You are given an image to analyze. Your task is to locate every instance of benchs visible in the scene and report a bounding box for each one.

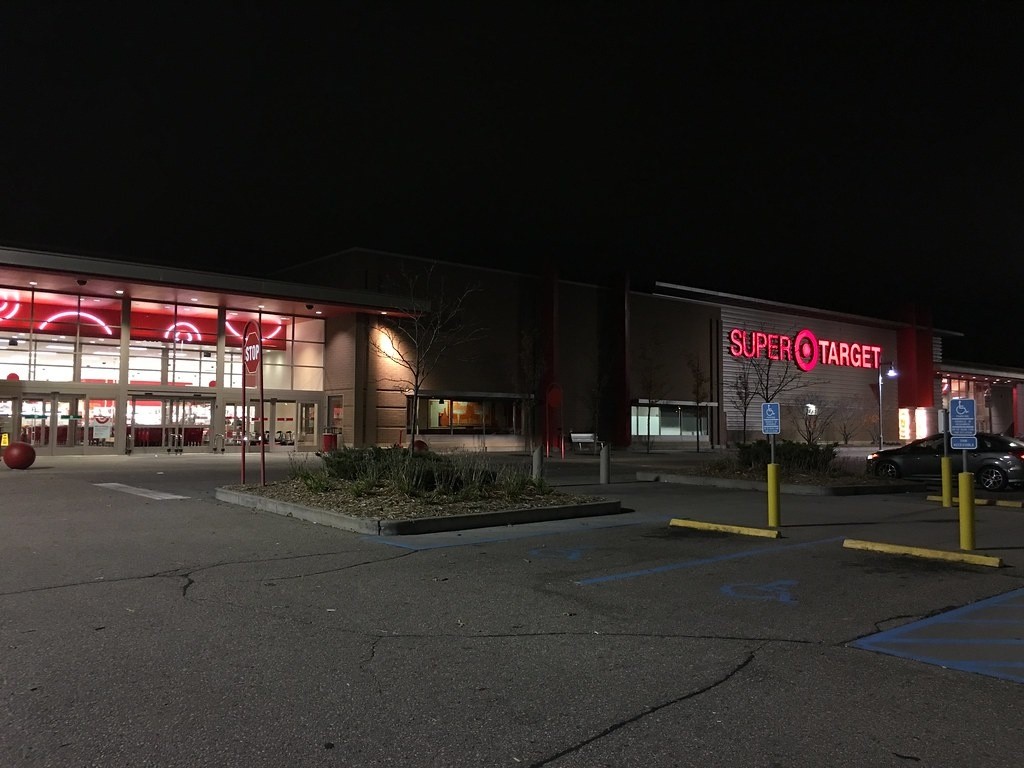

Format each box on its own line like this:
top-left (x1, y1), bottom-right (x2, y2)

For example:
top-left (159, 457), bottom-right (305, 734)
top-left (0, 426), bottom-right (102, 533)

top-left (570, 433), bottom-right (606, 453)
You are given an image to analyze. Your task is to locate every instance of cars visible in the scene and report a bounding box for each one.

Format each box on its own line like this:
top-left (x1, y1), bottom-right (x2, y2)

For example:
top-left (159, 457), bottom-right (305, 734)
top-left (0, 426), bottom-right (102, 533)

top-left (865, 431), bottom-right (1024, 492)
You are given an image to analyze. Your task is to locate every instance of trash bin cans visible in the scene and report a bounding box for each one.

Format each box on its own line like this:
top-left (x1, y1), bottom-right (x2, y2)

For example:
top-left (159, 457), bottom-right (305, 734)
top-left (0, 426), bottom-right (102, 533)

top-left (323, 426), bottom-right (339, 453)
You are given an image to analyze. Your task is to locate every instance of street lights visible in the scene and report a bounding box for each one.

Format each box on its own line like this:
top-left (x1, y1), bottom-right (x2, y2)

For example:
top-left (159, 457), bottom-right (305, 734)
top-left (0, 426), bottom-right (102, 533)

top-left (877, 361), bottom-right (898, 450)
